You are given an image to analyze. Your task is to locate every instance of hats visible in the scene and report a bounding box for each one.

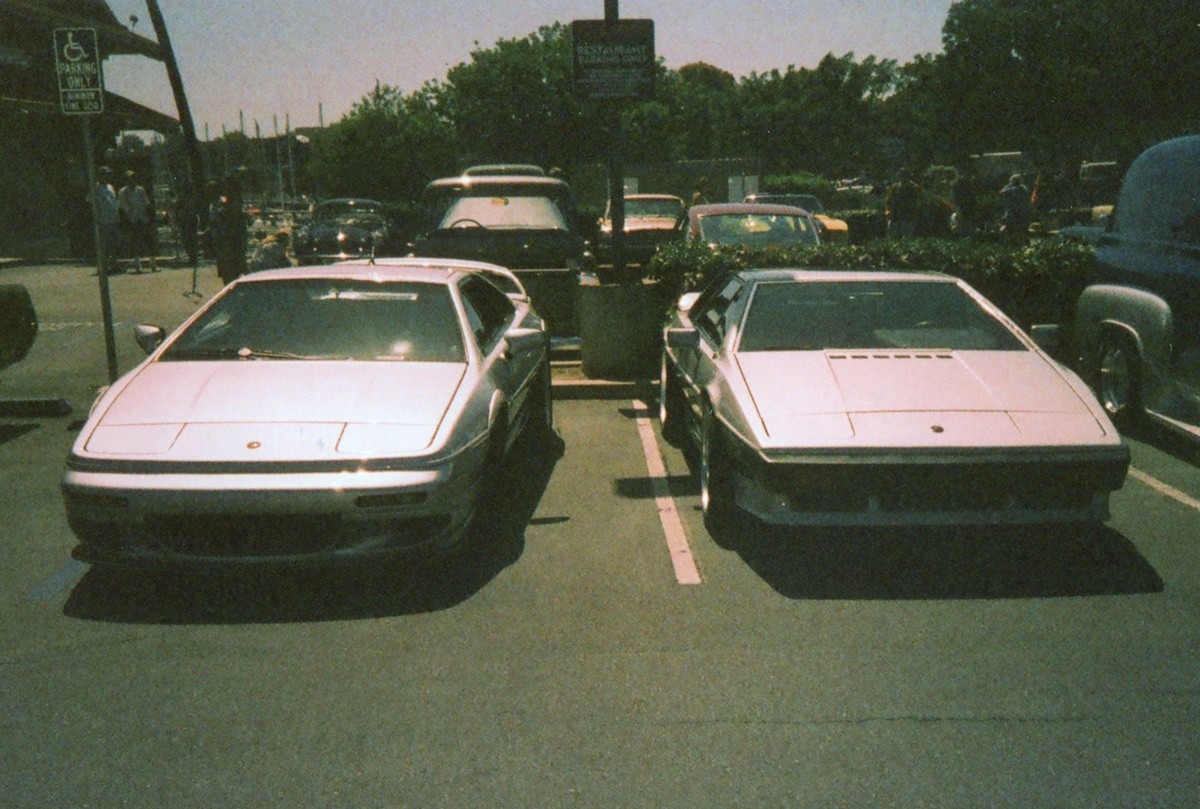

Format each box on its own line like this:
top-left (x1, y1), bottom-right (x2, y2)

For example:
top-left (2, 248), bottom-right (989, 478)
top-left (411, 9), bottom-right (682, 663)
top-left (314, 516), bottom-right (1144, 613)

top-left (96, 166), bottom-right (113, 177)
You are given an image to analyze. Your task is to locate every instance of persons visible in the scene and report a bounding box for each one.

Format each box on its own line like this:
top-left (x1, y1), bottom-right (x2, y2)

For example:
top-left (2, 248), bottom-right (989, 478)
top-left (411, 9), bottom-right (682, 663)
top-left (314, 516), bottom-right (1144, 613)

top-left (692, 175), bottom-right (713, 205)
top-left (117, 168), bottom-right (161, 274)
top-left (884, 162), bottom-right (1074, 239)
top-left (207, 198), bottom-right (292, 288)
top-left (85, 166), bottom-right (129, 276)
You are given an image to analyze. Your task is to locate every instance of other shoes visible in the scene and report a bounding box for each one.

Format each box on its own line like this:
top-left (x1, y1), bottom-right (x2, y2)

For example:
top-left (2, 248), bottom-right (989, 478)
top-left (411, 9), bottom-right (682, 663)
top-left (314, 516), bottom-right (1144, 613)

top-left (136, 267), bottom-right (142, 273)
top-left (151, 266), bottom-right (161, 272)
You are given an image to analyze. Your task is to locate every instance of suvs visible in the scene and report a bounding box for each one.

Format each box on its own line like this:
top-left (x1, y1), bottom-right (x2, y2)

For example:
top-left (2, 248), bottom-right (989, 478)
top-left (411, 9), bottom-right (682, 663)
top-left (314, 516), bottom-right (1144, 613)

top-left (1027, 134), bottom-right (1199, 443)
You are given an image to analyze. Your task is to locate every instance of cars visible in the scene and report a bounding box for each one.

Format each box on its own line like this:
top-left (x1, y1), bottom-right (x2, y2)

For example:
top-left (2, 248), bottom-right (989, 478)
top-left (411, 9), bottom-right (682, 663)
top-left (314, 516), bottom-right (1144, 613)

top-left (293, 199), bottom-right (408, 266)
top-left (413, 162), bottom-right (850, 286)
top-left (659, 268), bottom-right (1133, 550)
top-left (61, 262), bottom-right (550, 574)
